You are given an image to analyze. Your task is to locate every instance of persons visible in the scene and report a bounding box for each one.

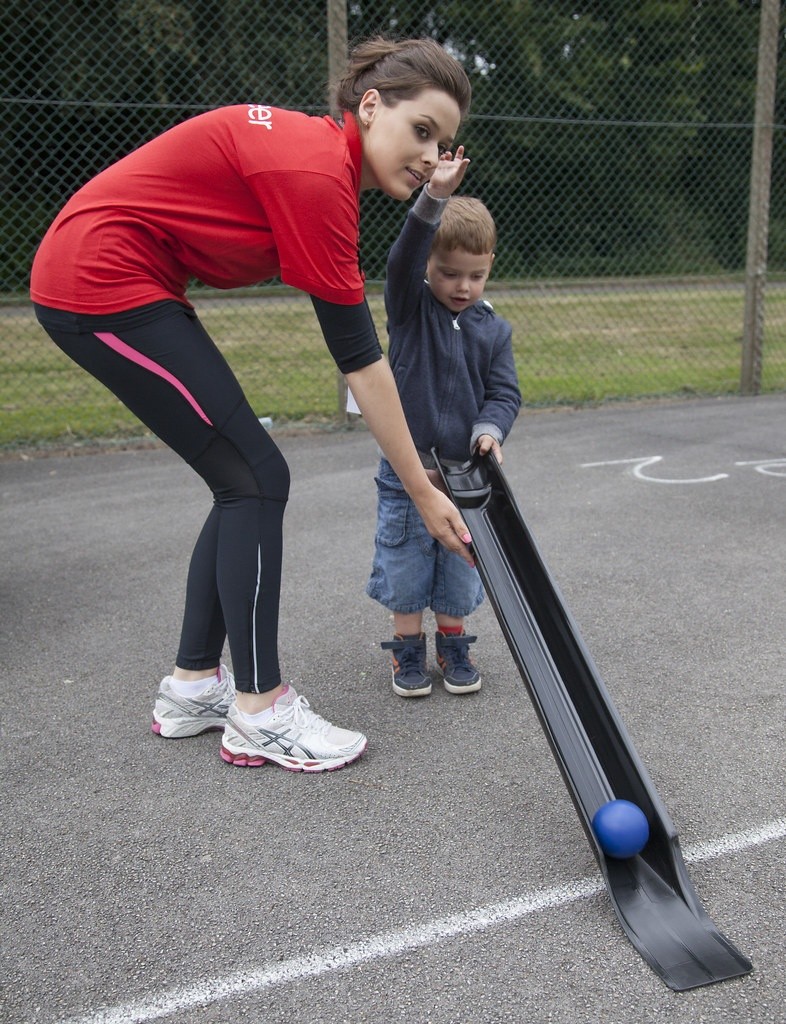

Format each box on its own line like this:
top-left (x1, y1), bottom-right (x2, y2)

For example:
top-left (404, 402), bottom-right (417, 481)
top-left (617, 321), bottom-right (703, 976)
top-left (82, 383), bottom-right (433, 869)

top-left (30, 38), bottom-right (472, 772)
top-left (365, 145), bottom-right (521, 696)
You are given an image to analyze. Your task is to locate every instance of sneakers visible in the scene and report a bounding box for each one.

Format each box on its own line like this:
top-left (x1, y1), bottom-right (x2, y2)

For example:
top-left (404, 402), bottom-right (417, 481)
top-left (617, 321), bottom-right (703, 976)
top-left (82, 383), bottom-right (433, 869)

top-left (433, 629), bottom-right (482, 694)
top-left (380, 633), bottom-right (433, 698)
top-left (151, 663), bottom-right (237, 739)
top-left (218, 685), bottom-right (368, 774)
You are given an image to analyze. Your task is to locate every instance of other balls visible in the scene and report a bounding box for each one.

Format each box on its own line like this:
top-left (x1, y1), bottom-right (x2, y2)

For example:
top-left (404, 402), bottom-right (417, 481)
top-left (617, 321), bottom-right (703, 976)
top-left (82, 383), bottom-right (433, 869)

top-left (592, 797), bottom-right (650, 859)
